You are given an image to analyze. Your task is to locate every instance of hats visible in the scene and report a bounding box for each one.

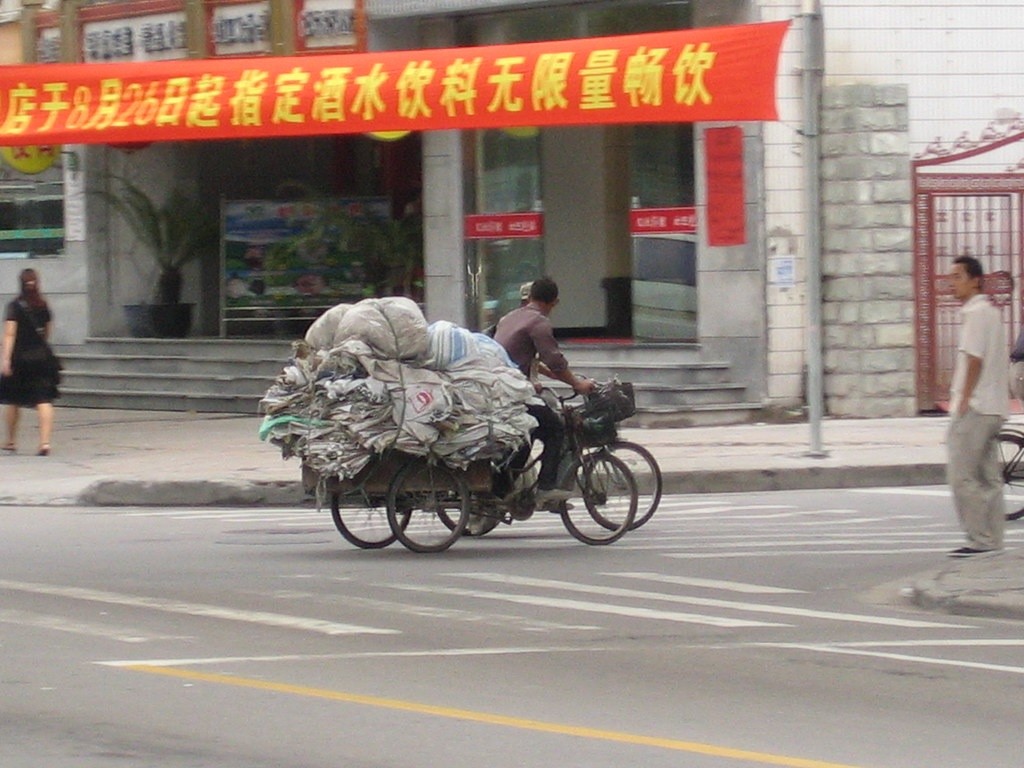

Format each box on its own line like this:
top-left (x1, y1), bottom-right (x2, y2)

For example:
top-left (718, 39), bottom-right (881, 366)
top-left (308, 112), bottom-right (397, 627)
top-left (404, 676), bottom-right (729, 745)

top-left (519, 281), bottom-right (534, 299)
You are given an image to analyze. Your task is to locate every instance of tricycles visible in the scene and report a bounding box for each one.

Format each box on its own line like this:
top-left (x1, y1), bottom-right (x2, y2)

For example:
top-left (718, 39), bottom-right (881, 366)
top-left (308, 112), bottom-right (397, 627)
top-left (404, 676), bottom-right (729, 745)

top-left (297, 370), bottom-right (639, 554)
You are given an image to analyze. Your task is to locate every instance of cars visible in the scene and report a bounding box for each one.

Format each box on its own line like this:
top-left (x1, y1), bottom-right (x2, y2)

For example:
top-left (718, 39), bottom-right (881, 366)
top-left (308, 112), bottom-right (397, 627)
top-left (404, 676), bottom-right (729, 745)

top-left (477, 233), bottom-right (699, 341)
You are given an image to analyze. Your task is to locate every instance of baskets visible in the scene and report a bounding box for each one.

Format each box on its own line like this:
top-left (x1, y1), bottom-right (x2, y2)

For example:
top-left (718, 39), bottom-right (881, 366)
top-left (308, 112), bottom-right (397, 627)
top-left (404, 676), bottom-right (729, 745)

top-left (587, 383), bottom-right (636, 421)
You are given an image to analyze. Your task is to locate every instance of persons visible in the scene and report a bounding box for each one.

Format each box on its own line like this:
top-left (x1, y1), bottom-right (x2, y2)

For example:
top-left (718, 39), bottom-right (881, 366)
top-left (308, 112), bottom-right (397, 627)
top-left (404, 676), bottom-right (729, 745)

top-left (488, 274), bottom-right (597, 511)
top-left (946, 254), bottom-right (1013, 557)
top-left (0, 268), bottom-right (63, 456)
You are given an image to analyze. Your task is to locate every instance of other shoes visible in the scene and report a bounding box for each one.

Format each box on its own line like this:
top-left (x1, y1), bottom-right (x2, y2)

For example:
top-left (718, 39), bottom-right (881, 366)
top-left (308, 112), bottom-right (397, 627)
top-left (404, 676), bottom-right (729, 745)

top-left (534, 488), bottom-right (572, 500)
top-left (947, 546), bottom-right (1006, 557)
top-left (535, 504), bottom-right (575, 511)
top-left (34, 443), bottom-right (52, 456)
top-left (3, 443), bottom-right (18, 451)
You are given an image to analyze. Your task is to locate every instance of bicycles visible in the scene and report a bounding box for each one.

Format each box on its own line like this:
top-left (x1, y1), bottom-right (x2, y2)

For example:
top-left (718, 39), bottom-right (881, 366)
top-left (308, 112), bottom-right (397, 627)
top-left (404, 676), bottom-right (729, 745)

top-left (994, 420), bottom-right (1024, 521)
top-left (435, 386), bottom-right (664, 538)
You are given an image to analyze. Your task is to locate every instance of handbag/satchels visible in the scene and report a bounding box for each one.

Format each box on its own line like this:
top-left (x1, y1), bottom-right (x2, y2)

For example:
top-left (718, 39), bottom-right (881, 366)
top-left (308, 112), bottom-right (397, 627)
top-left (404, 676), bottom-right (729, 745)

top-left (570, 396), bottom-right (618, 447)
top-left (46, 350), bottom-right (63, 385)
